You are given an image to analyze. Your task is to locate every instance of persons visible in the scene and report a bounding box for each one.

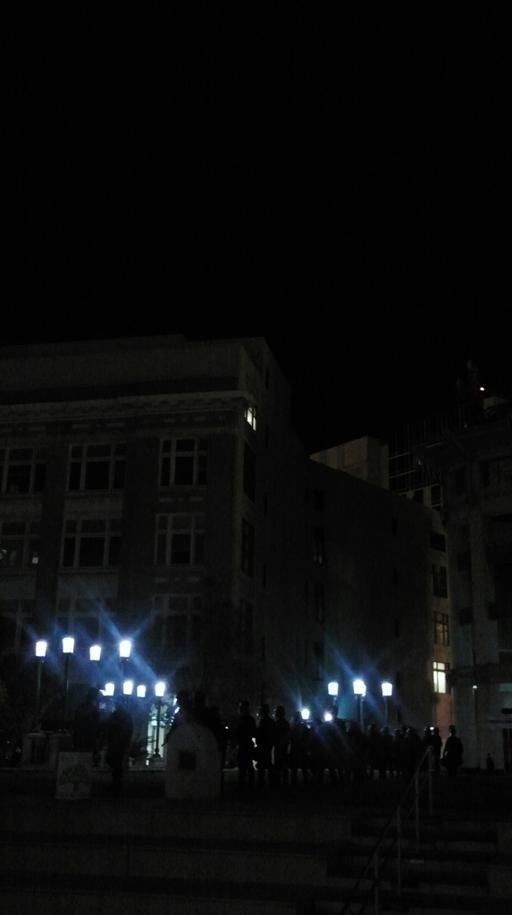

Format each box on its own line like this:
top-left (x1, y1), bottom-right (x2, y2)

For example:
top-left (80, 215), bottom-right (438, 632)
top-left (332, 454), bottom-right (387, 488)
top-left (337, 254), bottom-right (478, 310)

top-left (229, 701), bottom-right (463, 792)
top-left (486, 753), bottom-right (495, 771)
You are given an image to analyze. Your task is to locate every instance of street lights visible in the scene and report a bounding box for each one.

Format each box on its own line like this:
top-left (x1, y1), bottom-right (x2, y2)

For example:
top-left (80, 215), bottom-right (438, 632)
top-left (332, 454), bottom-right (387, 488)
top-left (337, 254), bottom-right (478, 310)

top-left (328, 680), bottom-right (339, 719)
top-left (353, 679), bottom-right (367, 726)
top-left (63, 636), bottom-right (74, 728)
top-left (381, 681), bottom-right (393, 725)
top-left (90, 637), bottom-right (165, 757)
top-left (35, 638), bottom-right (48, 729)
